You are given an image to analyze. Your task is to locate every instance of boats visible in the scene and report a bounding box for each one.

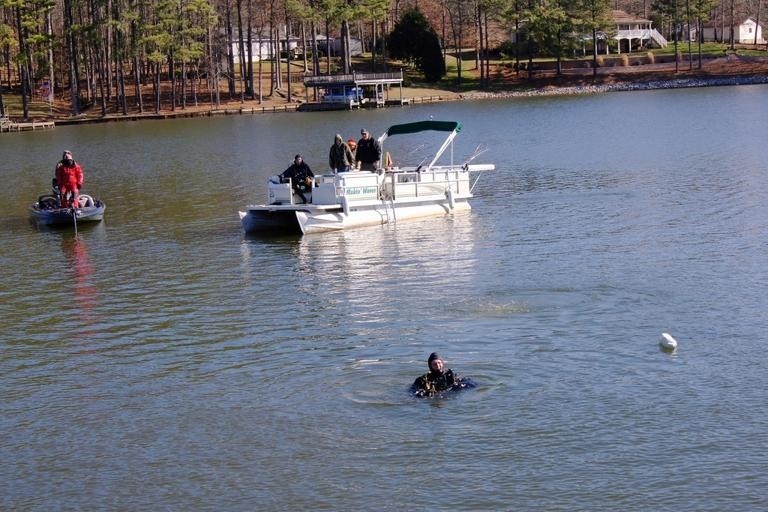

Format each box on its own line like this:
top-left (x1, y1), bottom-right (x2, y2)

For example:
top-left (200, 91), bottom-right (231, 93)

top-left (26, 193), bottom-right (109, 228)
top-left (236, 118), bottom-right (493, 239)
top-left (320, 86), bottom-right (366, 108)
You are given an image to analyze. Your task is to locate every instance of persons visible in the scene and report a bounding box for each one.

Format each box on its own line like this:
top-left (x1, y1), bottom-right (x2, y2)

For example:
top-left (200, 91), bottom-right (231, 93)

top-left (53, 149), bottom-right (84, 211)
top-left (326, 133), bottom-right (355, 174)
top-left (354, 127), bottom-right (381, 173)
top-left (279, 152), bottom-right (319, 204)
top-left (346, 137), bottom-right (358, 157)
top-left (57, 240), bottom-right (107, 354)
top-left (407, 351), bottom-right (462, 400)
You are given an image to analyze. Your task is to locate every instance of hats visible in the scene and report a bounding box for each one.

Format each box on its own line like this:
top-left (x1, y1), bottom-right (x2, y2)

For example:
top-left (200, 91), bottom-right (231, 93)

top-left (360, 127), bottom-right (368, 133)
top-left (428, 351), bottom-right (444, 376)
top-left (347, 139), bottom-right (355, 145)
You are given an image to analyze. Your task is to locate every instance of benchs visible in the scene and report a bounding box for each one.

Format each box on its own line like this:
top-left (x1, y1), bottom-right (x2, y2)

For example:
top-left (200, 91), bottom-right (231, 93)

top-left (268, 171), bottom-right (380, 205)
